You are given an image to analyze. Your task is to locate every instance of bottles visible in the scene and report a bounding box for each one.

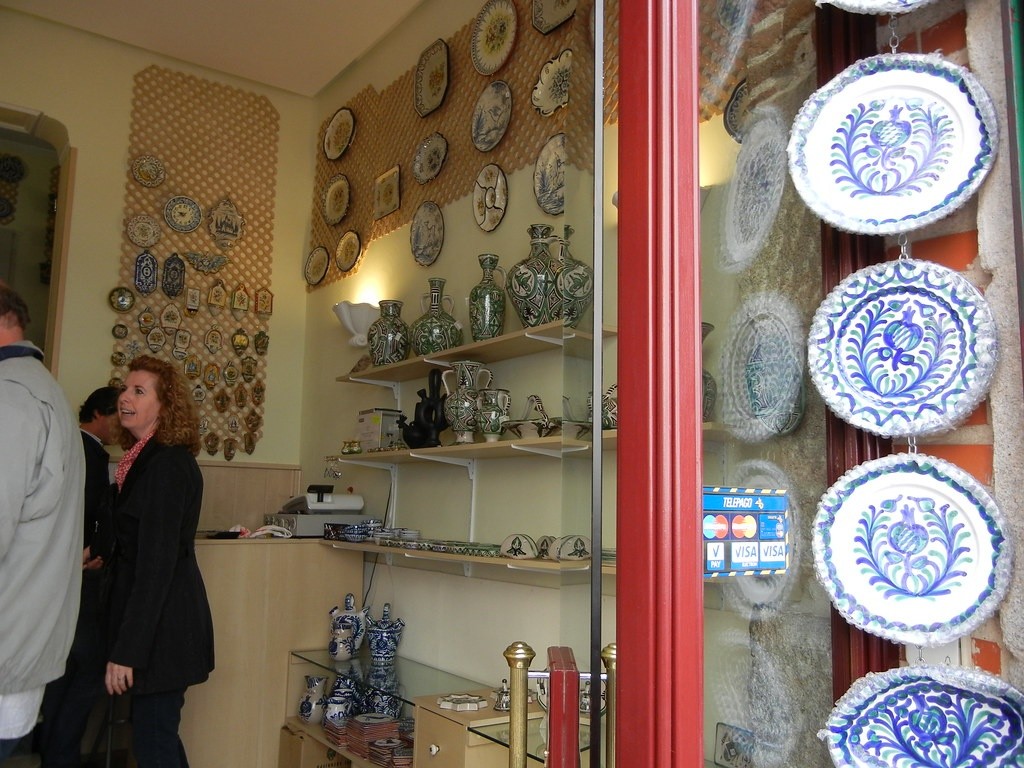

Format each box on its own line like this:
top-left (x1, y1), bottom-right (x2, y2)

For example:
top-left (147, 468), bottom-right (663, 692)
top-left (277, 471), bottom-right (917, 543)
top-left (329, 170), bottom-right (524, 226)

top-left (410, 278), bottom-right (463, 357)
top-left (368, 300), bottom-right (410, 367)
top-left (341, 440), bottom-right (361, 454)
top-left (298, 592), bottom-right (405, 724)
top-left (469, 254), bottom-right (507, 341)
top-left (441, 359), bottom-right (511, 443)
top-left (506, 224), bottom-right (594, 329)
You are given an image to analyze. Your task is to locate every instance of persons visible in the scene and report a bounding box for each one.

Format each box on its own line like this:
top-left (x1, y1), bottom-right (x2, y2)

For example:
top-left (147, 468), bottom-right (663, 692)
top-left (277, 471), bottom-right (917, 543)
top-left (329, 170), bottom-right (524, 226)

top-left (35, 385), bottom-right (126, 768)
top-left (83, 356), bottom-right (215, 768)
top-left (0, 278), bottom-right (88, 768)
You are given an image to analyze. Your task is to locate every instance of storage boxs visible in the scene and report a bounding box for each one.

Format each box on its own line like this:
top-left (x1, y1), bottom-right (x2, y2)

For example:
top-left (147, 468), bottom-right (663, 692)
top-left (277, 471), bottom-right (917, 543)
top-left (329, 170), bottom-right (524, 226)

top-left (277, 724), bottom-right (351, 768)
top-left (355, 406), bottom-right (402, 451)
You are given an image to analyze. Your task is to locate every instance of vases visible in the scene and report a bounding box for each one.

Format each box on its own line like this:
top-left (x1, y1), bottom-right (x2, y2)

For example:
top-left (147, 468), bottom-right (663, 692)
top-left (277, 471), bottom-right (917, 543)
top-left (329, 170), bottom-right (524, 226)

top-left (701, 321), bottom-right (717, 422)
top-left (560, 225), bottom-right (593, 330)
top-left (474, 386), bottom-right (511, 444)
top-left (332, 687), bottom-right (361, 717)
top-left (323, 697), bottom-right (353, 725)
top-left (365, 300), bottom-right (411, 367)
top-left (407, 277), bottom-right (464, 357)
top-left (296, 674), bottom-right (330, 725)
top-left (442, 359), bottom-right (494, 445)
top-left (468, 253), bottom-right (506, 341)
top-left (506, 222), bottom-right (563, 330)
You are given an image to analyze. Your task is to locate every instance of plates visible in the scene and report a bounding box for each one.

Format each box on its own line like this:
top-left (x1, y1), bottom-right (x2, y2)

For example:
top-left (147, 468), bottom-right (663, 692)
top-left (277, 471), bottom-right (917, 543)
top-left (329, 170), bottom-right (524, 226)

top-left (164, 196), bottom-right (203, 233)
top-left (127, 214), bottom-right (160, 247)
top-left (106, 285), bottom-right (135, 313)
top-left (304, 38), bottom-right (450, 285)
top-left (470, 0), bottom-right (578, 233)
top-left (132, 156), bottom-right (165, 187)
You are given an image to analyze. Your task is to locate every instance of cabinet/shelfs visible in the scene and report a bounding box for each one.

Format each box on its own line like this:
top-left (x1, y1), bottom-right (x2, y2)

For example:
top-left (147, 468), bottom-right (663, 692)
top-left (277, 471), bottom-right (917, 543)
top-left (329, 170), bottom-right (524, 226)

top-left (600, 420), bottom-right (727, 590)
top-left (562, 327), bottom-right (602, 587)
top-left (325, 320), bottom-right (562, 589)
top-left (602, 322), bottom-right (616, 579)
top-left (287, 648), bottom-right (591, 768)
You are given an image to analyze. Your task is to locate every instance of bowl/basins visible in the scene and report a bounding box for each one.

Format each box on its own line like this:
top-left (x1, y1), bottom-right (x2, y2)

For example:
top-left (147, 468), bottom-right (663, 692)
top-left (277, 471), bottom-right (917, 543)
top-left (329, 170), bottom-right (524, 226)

top-left (323, 519), bottom-right (501, 558)
